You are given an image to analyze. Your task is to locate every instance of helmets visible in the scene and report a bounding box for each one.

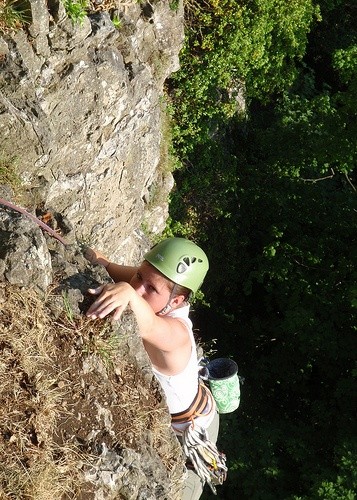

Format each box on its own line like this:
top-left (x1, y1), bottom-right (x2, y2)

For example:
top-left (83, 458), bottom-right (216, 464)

top-left (143, 237), bottom-right (209, 299)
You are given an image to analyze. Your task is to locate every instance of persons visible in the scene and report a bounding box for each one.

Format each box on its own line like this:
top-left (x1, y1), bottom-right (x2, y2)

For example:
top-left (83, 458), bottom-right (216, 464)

top-left (81, 237), bottom-right (220, 488)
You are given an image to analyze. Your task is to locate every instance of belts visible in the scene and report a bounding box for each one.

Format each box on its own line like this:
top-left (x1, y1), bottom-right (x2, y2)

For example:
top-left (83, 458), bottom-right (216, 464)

top-left (169, 385), bottom-right (210, 425)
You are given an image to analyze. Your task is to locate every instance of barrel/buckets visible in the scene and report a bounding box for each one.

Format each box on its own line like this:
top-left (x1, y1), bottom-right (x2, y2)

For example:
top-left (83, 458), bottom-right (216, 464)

top-left (205, 357), bottom-right (241, 414)
